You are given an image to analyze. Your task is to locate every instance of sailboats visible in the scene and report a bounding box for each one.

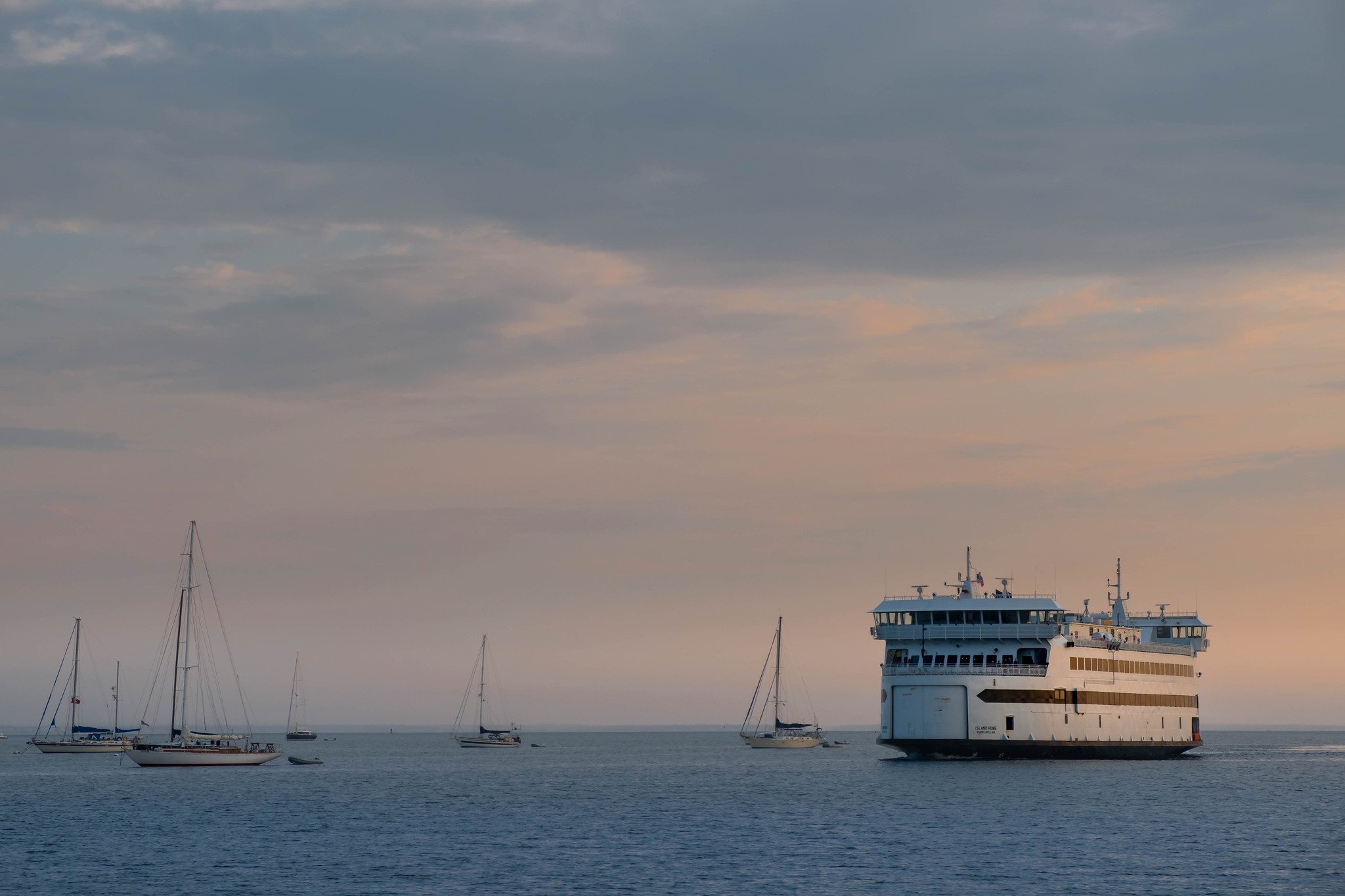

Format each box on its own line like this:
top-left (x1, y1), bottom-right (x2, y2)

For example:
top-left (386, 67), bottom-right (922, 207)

top-left (739, 609), bottom-right (829, 748)
top-left (119, 516), bottom-right (283, 767)
top-left (447, 633), bottom-right (523, 748)
top-left (11, 616), bottom-right (141, 755)
top-left (284, 650), bottom-right (318, 742)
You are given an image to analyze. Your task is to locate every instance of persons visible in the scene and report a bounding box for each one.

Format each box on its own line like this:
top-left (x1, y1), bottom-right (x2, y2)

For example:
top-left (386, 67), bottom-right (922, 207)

top-left (896, 650), bottom-right (902, 664)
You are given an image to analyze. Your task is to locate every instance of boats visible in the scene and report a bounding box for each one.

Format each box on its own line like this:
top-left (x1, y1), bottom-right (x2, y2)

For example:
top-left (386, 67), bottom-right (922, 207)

top-left (287, 755), bottom-right (325, 765)
top-left (324, 738), bottom-right (337, 741)
top-left (865, 545), bottom-right (1215, 762)
top-left (0, 733), bottom-right (8, 739)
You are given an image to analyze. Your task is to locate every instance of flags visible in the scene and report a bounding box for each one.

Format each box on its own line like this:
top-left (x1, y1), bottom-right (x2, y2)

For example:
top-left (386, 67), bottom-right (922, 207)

top-left (977, 574), bottom-right (984, 587)
top-left (70, 697), bottom-right (80, 704)
top-left (141, 720), bottom-right (150, 727)
